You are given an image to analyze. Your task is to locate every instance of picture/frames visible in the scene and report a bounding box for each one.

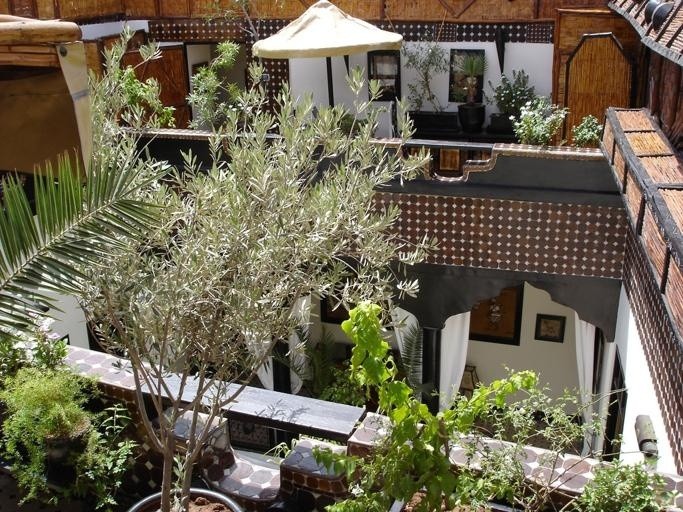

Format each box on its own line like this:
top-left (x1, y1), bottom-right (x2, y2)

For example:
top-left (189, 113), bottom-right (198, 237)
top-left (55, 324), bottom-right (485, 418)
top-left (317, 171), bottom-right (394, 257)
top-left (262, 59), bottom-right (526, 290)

top-left (534, 313), bottom-right (566, 344)
top-left (468, 281), bottom-right (523, 347)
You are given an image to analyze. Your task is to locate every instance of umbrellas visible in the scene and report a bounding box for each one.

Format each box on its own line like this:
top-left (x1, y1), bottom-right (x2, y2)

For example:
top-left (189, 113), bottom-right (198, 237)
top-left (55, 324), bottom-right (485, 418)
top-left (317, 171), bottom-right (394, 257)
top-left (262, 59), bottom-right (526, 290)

top-left (250, 1), bottom-right (404, 118)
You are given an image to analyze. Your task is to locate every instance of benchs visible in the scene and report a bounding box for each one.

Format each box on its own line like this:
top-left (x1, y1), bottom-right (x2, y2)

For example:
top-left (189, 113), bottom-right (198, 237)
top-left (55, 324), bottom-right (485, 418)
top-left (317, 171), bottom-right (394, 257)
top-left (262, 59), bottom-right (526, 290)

top-left (405, 111), bottom-right (517, 142)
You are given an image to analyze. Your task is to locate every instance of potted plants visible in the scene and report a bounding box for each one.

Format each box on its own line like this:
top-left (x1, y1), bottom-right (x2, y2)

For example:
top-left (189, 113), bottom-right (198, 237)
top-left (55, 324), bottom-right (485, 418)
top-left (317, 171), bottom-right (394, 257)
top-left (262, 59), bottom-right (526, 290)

top-left (456, 51), bottom-right (489, 132)
top-left (74, 62), bottom-right (432, 512)
top-left (311, 297), bottom-right (524, 512)
